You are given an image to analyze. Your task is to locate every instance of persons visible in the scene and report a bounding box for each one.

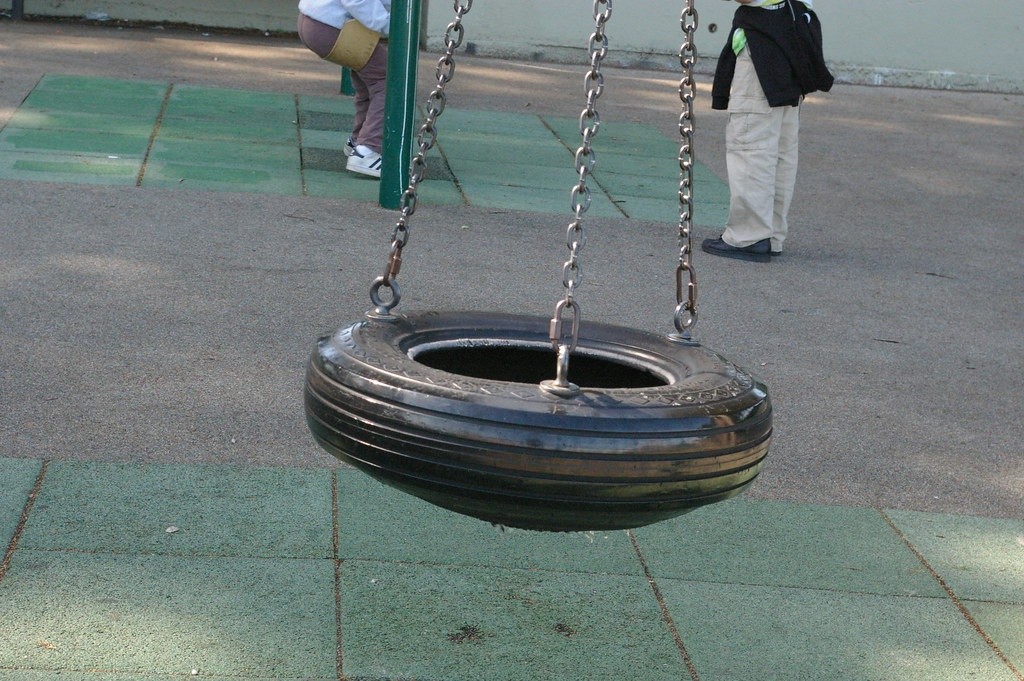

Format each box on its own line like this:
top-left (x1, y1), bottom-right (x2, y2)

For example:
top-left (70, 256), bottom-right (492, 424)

top-left (701, 0), bottom-right (835, 264)
top-left (297, 0), bottom-right (392, 179)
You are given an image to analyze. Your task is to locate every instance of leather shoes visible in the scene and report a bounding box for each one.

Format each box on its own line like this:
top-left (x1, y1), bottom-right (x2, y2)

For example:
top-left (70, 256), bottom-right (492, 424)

top-left (701, 234), bottom-right (772, 263)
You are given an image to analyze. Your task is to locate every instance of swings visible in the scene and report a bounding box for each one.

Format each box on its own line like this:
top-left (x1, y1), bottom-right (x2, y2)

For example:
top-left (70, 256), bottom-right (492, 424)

top-left (295, 1), bottom-right (777, 537)
top-left (319, 18), bottom-right (382, 73)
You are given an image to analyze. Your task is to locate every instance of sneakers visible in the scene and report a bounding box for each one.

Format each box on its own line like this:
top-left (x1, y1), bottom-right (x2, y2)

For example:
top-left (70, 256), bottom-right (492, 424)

top-left (343, 135), bottom-right (359, 157)
top-left (345, 141), bottom-right (382, 177)
